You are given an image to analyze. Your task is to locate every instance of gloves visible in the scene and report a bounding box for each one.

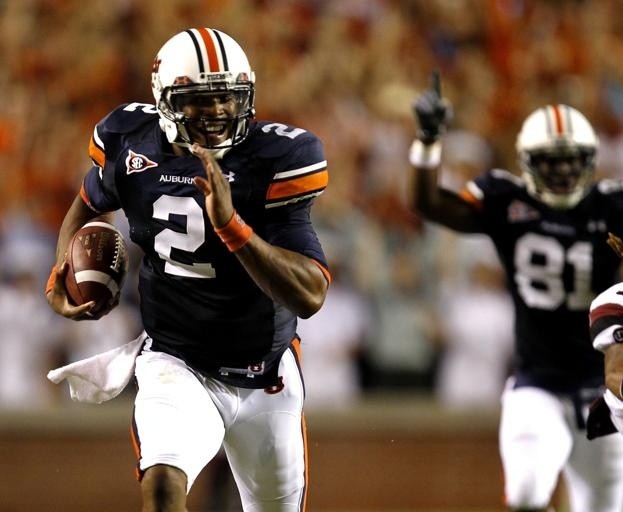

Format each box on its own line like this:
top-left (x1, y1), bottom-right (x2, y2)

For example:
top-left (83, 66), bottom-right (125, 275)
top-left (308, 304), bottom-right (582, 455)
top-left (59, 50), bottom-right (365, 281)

top-left (412, 68), bottom-right (452, 145)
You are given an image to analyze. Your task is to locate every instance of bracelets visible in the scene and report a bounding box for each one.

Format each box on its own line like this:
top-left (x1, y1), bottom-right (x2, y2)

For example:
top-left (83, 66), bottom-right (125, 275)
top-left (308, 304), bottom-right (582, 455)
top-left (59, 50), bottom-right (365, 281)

top-left (214, 210), bottom-right (254, 252)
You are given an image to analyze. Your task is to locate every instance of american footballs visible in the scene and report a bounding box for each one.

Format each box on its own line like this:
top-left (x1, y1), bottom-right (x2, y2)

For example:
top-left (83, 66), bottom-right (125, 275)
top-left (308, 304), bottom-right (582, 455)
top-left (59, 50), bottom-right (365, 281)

top-left (64, 216), bottom-right (130, 319)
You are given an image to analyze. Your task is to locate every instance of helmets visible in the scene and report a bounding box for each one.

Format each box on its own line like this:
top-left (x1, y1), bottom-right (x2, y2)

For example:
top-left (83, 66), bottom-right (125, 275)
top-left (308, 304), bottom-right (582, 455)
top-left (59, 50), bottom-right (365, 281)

top-left (150, 26), bottom-right (258, 152)
top-left (514, 103), bottom-right (600, 209)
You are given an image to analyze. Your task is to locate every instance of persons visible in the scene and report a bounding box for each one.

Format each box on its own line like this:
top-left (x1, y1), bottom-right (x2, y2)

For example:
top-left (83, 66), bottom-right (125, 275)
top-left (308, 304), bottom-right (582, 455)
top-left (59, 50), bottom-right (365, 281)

top-left (395, 64), bottom-right (622, 509)
top-left (42, 23), bottom-right (328, 512)
top-left (0, 0), bottom-right (623, 403)
